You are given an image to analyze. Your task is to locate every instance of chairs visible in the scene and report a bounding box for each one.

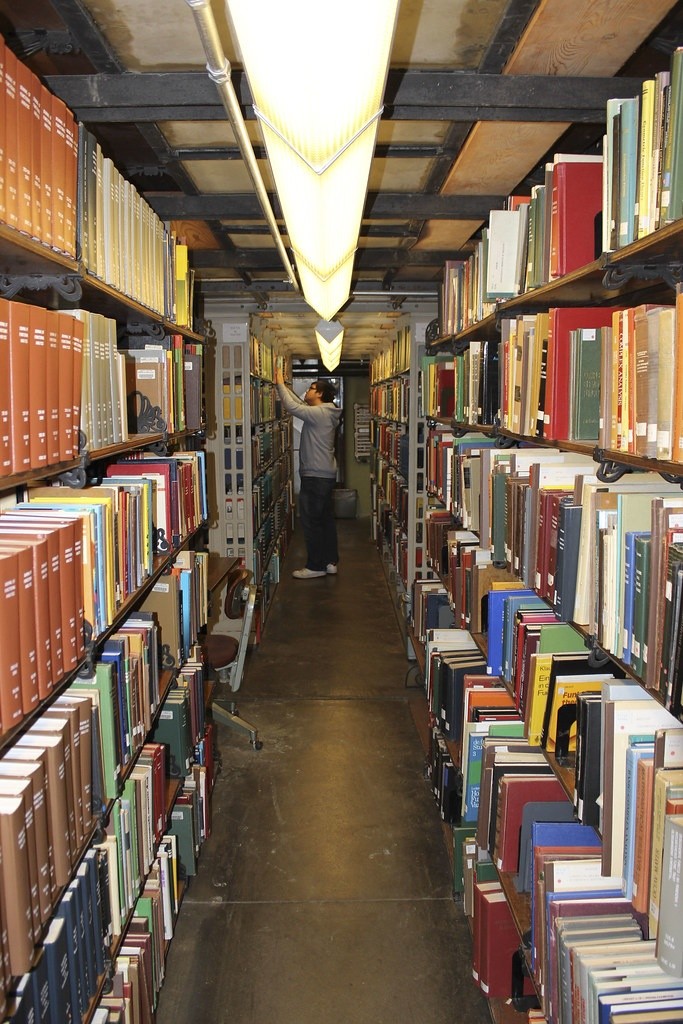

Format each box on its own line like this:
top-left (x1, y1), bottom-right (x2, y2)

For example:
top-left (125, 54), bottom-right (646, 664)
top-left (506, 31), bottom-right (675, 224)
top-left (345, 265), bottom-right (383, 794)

top-left (202, 564), bottom-right (262, 749)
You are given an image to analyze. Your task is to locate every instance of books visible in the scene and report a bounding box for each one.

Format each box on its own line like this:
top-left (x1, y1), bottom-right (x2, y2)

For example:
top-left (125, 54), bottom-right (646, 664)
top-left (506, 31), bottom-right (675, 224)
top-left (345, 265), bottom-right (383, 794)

top-left (0, 32), bottom-right (293, 1024)
top-left (371, 48), bottom-right (683, 1024)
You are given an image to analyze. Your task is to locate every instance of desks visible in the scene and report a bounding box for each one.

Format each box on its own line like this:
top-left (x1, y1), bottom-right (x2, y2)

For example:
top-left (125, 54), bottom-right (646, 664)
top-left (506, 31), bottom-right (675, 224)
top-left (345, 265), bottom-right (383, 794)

top-left (206, 556), bottom-right (240, 594)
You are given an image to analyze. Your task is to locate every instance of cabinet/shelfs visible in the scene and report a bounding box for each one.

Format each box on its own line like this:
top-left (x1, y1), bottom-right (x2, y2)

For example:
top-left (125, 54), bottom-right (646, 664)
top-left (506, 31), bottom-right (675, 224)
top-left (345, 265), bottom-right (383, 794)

top-left (210, 310), bottom-right (295, 646)
top-left (366, 311), bottom-right (440, 658)
top-left (424, 217), bottom-right (683, 1024)
top-left (0, 221), bottom-right (219, 1023)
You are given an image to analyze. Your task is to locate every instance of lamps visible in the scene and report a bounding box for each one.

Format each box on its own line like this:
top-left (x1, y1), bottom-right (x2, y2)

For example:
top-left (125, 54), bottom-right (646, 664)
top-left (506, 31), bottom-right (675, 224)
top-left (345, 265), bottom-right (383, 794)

top-left (314, 316), bottom-right (344, 372)
top-left (223, 0), bottom-right (396, 321)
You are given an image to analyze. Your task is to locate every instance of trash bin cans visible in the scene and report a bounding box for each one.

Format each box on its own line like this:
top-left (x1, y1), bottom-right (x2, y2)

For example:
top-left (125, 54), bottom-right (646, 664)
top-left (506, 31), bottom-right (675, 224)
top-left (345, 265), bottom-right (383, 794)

top-left (332, 487), bottom-right (359, 520)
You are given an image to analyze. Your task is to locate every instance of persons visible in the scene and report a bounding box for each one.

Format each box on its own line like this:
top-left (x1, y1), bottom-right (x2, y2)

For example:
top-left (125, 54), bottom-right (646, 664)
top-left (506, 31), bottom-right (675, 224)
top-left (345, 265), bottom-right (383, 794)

top-left (277, 367), bottom-right (343, 578)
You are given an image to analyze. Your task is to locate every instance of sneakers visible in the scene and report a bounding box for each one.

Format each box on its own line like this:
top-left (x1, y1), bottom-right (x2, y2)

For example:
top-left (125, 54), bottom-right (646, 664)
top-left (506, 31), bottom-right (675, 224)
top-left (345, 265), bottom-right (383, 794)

top-left (292, 568), bottom-right (326, 579)
top-left (326, 564), bottom-right (337, 573)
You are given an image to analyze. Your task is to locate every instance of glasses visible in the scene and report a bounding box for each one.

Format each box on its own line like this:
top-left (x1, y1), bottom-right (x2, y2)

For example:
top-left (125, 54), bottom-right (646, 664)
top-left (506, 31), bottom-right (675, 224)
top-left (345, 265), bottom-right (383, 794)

top-left (309, 387), bottom-right (317, 391)
